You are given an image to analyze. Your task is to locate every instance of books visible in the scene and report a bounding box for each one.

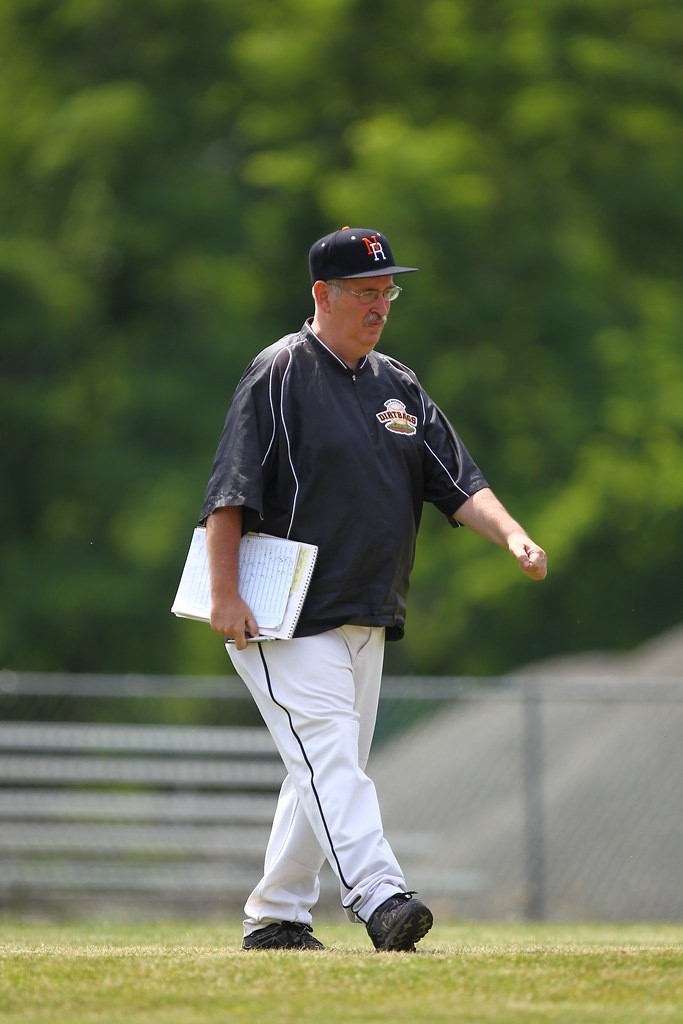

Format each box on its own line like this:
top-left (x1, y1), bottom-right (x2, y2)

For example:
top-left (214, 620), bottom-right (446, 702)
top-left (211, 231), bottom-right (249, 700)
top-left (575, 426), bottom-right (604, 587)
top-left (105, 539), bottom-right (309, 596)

top-left (171, 526), bottom-right (318, 641)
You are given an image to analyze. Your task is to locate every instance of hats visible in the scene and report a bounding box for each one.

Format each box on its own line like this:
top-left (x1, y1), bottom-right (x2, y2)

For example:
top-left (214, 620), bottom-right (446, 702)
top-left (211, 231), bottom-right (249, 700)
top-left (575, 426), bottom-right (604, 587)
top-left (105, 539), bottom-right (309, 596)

top-left (309, 226), bottom-right (419, 285)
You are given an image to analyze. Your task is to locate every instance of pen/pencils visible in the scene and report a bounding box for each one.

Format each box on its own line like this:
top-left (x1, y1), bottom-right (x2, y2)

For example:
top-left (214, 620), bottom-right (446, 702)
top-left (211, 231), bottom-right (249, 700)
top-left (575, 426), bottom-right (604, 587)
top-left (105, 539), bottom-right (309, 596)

top-left (225, 637), bottom-right (280, 642)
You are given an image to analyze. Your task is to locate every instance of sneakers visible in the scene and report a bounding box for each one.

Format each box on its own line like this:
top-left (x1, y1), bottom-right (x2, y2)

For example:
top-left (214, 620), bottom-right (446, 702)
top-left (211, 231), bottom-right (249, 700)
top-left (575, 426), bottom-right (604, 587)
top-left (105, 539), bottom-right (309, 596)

top-left (364, 890), bottom-right (433, 952)
top-left (241, 921), bottom-right (326, 950)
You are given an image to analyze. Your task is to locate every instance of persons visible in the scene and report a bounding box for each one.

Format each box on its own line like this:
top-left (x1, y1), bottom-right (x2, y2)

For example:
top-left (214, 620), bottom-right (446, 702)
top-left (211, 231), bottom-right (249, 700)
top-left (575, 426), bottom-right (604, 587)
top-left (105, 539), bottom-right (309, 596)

top-left (197, 226), bottom-right (549, 953)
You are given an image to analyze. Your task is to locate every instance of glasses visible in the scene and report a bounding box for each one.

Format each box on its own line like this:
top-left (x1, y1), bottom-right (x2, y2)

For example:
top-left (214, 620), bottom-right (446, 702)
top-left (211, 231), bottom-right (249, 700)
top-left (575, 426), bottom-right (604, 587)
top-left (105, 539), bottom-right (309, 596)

top-left (326, 283), bottom-right (403, 304)
